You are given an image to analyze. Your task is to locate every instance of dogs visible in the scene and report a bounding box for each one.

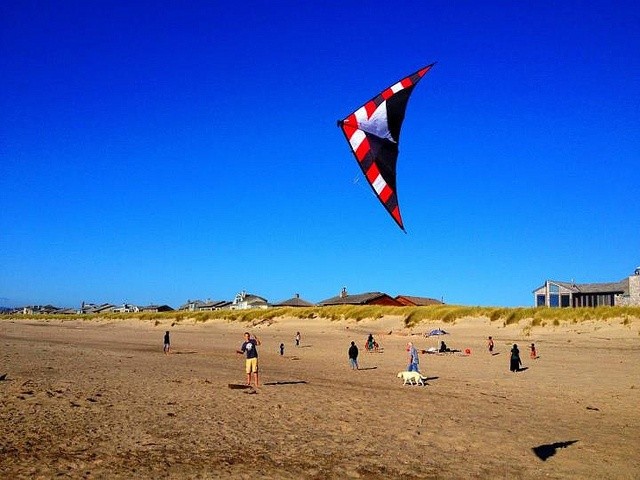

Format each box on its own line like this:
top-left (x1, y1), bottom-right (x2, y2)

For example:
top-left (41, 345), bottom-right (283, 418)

top-left (397, 371), bottom-right (428, 386)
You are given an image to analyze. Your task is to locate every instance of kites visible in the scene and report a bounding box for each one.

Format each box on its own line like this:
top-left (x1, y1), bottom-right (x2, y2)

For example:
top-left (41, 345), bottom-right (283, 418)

top-left (337, 61), bottom-right (437, 236)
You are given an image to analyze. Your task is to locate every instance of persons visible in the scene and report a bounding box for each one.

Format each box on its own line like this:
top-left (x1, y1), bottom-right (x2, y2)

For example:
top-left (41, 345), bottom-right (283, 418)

top-left (439, 341), bottom-right (447, 352)
top-left (406, 343), bottom-right (419, 374)
top-left (295, 332), bottom-right (301, 346)
top-left (487, 336), bottom-right (495, 353)
top-left (236, 333), bottom-right (260, 388)
top-left (162, 331), bottom-right (170, 354)
top-left (348, 341), bottom-right (359, 370)
top-left (510, 344), bottom-right (522, 372)
top-left (278, 343), bottom-right (285, 355)
top-left (365, 333), bottom-right (379, 353)
top-left (528, 343), bottom-right (536, 359)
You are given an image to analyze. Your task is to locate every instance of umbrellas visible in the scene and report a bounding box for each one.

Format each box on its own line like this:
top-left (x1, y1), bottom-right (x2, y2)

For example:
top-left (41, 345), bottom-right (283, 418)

top-left (429, 328), bottom-right (449, 354)
